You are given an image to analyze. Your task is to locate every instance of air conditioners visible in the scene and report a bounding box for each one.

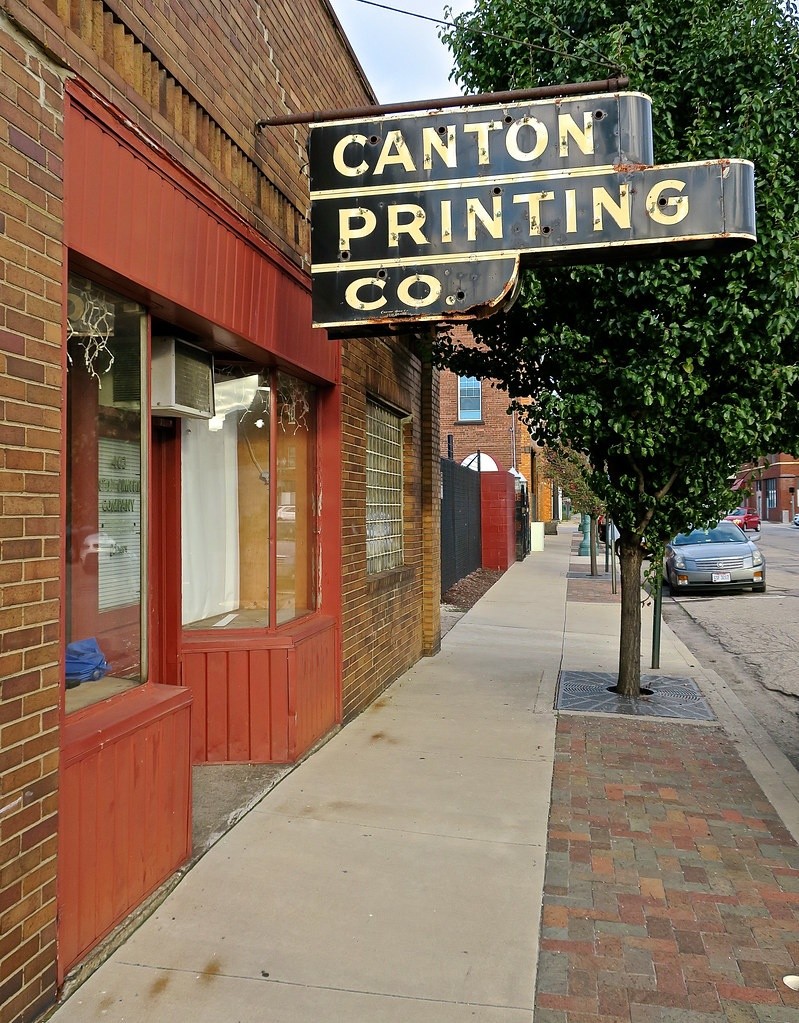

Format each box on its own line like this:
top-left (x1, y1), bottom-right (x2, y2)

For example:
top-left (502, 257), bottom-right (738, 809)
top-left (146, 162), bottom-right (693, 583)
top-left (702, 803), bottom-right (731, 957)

top-left (99, 332), bottom-right (216, 420)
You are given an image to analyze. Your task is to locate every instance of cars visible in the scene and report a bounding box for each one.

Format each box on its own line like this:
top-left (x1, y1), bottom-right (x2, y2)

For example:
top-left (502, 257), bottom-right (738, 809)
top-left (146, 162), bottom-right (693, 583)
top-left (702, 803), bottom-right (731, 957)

top-left (663, 520), bottom-right (766, 596)
top-left (724, 506), bottom-right (761, 532)
top-left (794, 513), bottom-right (799, 526)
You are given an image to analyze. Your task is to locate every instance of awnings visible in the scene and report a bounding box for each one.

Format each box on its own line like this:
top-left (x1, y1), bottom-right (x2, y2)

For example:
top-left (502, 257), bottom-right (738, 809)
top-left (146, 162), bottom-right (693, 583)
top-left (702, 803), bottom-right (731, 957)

top-left (730, 479), bottom-right (745, 491)
